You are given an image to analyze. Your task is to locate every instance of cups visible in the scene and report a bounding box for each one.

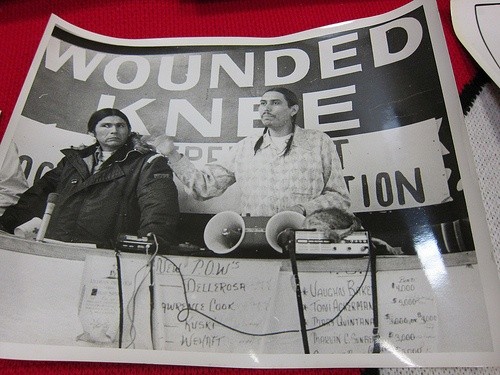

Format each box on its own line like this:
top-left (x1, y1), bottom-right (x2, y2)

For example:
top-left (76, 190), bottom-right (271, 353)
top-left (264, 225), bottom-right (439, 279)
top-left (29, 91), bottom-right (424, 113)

top-left (418, 218), bottom-right (475, 256)
top-left (13, 217), bottom-right (42, 239)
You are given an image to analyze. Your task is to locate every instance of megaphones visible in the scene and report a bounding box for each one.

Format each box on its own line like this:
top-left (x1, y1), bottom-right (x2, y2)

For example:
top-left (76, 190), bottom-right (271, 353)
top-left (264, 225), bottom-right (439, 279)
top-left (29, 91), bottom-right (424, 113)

top-left (203, 212), bottom-right (245, 254)
top-left (265, 211), bottom-right (308, 253)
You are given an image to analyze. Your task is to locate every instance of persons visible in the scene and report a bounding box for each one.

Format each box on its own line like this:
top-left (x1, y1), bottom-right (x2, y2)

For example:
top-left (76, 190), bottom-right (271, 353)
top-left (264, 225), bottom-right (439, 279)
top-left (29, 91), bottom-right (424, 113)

top-left (0, 107), bottom-right (179, 247)
top-left (156, 86), bottom-right (353, 217)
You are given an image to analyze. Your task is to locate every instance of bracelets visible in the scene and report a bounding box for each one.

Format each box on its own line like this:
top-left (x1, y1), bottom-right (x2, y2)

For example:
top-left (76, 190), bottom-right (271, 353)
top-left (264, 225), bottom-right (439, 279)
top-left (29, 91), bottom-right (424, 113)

top-left (294, 204), bottom-right (306, 216)
